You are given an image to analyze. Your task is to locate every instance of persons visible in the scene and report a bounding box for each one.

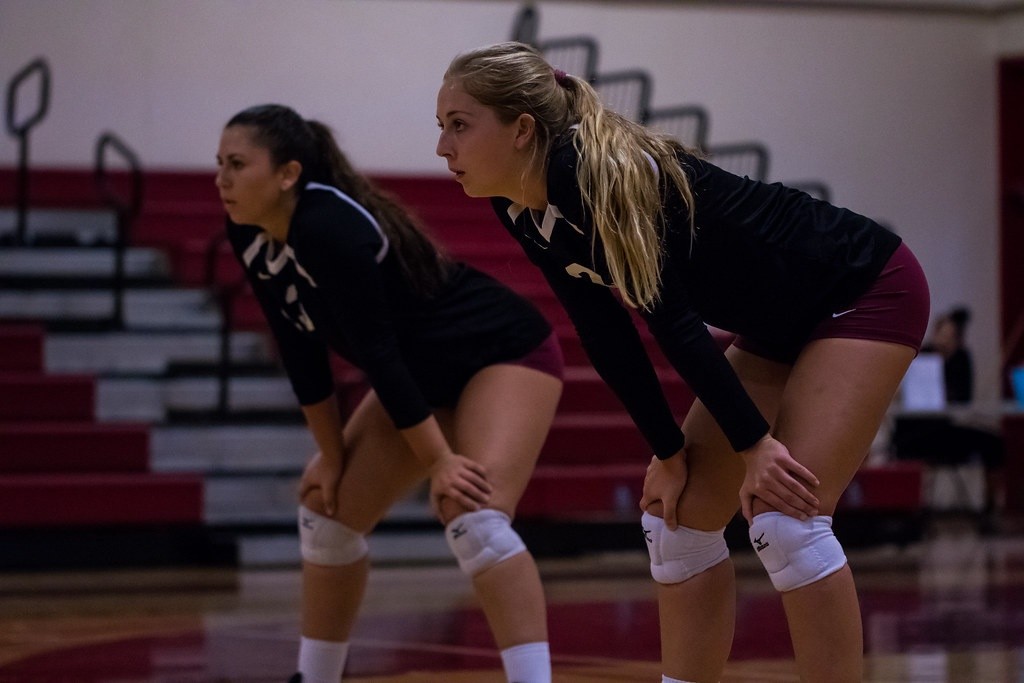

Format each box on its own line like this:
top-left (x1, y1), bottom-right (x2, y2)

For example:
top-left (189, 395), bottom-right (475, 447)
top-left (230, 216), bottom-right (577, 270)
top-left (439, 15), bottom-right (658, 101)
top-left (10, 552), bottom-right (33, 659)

top-left (436, 42), bottom-right (931, 683)
top-left (216, 105), bottom-right (565, 683)
top-left (918, 310), bottom-right (974, 407)
